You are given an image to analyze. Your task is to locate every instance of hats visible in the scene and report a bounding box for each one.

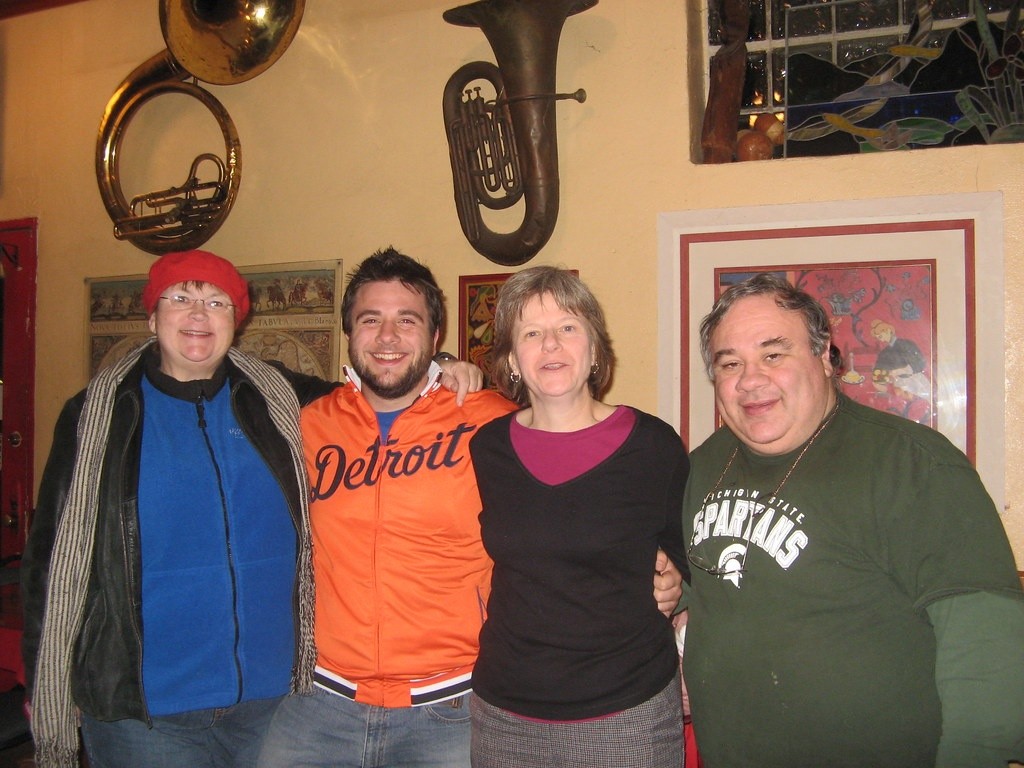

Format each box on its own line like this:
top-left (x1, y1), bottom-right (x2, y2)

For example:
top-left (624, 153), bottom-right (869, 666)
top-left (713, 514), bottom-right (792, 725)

top-left (144, 250), bottom-right (250, 330)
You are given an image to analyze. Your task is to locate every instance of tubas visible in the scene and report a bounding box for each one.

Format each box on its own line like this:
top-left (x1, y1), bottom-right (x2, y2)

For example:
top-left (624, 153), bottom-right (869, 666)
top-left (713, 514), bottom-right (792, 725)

top-left (442, 0), bottom-right (600, 267)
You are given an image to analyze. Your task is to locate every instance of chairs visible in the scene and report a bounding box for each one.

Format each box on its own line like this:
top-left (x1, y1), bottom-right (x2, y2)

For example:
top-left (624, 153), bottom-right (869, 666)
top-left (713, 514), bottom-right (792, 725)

top-left (0, 554), bottom-right (84, 768)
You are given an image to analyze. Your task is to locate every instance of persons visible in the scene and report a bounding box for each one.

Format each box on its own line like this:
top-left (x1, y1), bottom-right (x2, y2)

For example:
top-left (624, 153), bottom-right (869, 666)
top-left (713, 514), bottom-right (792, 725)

top-left (670, 273), bottom-right (1024, 768)
top-left (468, 266), bottom-right (691, 768)
top-left (256, 245), bottom-right (682, 768)
top-left (20, 251), bottom-right (482, 768)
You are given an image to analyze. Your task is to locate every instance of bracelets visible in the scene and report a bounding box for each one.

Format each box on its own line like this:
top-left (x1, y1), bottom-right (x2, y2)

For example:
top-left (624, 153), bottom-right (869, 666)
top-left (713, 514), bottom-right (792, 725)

top-left (441, 357), bottom-right (452, 360)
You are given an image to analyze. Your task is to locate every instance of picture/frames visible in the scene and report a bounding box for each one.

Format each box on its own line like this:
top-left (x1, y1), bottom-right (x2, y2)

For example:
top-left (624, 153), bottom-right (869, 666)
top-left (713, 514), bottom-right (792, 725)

top-left (657, 192), bottom-right (1006, 515)
top-left (459, 270), bottom-right (578, 406)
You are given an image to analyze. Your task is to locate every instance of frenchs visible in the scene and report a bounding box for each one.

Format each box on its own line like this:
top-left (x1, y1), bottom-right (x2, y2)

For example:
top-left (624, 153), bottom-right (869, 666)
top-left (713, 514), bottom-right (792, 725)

top-left (95, 0), bottom-right (306, 257)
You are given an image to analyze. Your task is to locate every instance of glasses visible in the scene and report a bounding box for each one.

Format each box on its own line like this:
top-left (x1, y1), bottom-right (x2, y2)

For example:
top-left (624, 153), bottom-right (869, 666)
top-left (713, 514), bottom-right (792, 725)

top-left (687, 482), bottom-right (774, 577)
top-left (160, 295), bottom-right (236, 314)
